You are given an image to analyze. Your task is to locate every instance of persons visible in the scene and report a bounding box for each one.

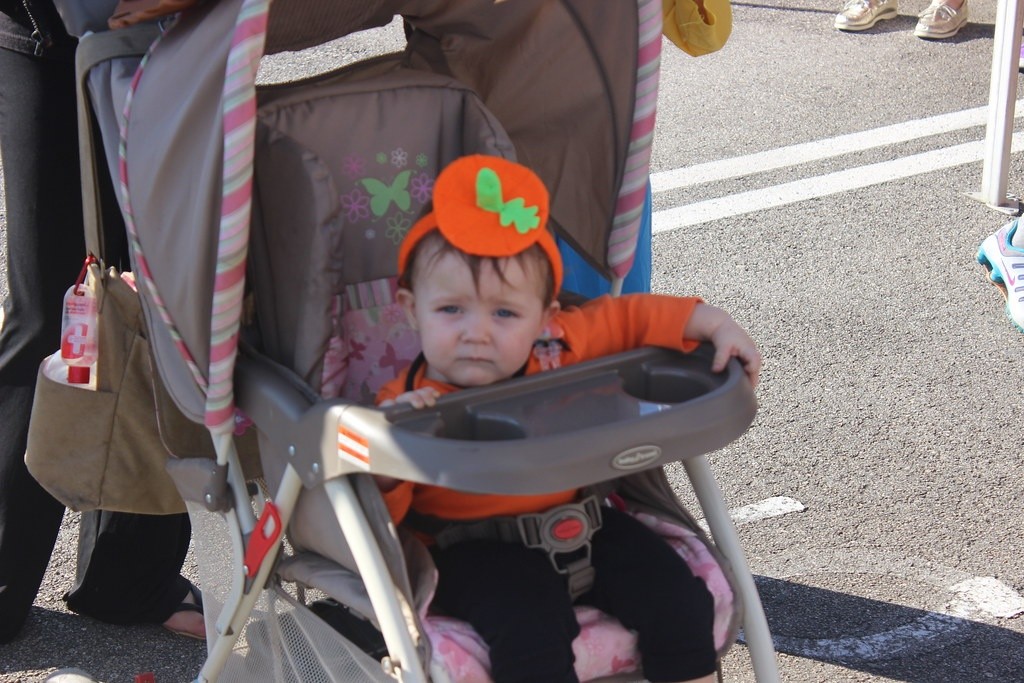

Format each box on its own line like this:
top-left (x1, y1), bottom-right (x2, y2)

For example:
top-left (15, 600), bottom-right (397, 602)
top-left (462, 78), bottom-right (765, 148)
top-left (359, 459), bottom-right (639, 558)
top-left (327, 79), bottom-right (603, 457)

top-left (2, 0), bottom-right (226, 656)
top-left (977, 208), bottom-right (1024, 336)
top-left (374, 157), bottom-right (762, 681)
top-left (834, 1), bottom-right (970, 38)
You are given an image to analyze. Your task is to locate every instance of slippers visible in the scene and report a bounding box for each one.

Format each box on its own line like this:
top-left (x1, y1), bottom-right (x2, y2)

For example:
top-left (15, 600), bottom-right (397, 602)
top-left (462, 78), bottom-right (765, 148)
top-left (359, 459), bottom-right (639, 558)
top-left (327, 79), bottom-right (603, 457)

top-left (160, 583), bottom-right (223, 640)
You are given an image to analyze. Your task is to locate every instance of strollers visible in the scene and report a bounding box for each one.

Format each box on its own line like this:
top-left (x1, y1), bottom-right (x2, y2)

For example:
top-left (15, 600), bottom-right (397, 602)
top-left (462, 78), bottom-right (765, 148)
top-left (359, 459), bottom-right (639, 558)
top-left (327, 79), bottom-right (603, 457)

top-left (55, 0), bottom-right (784, 682)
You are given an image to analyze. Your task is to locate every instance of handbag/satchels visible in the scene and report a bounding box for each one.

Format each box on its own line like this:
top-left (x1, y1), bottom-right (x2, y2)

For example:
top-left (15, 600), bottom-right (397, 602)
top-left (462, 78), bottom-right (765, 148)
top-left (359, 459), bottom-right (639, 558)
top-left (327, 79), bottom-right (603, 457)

top-left (23, 264), bottom-right (264, 515)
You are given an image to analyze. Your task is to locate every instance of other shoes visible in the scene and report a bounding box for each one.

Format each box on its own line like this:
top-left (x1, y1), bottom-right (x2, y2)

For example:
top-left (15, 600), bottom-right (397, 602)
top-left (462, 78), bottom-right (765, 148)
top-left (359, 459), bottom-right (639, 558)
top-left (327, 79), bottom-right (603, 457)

top-left (1019, 42), bottom-right (1024, 69)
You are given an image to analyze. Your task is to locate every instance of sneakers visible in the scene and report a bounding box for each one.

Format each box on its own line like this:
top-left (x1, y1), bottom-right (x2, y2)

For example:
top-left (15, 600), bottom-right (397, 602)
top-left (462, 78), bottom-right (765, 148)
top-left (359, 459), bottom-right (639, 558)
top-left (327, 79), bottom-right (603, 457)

top-left (977, 220), bottom-right (1024, 333)
top-left (913, 0), bottom-right (967, 38)
top-left (834, 0), bottom-right (897, 30)
